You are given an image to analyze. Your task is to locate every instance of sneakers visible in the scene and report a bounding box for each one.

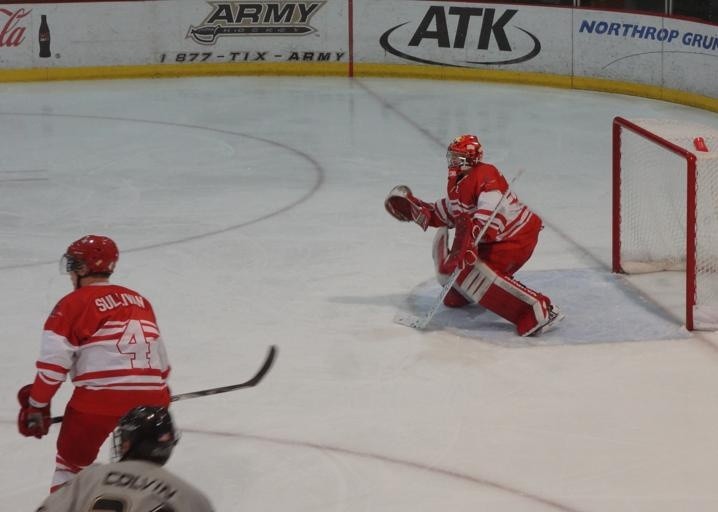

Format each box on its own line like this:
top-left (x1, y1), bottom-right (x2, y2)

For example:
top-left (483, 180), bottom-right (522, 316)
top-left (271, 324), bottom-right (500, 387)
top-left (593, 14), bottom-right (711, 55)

top-left (517, 292), bottom-right (553, 336)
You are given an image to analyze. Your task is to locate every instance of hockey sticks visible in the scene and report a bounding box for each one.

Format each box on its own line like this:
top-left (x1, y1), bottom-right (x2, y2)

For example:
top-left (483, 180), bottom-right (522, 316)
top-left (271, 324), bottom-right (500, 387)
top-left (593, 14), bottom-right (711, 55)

top-left (392, 166), bottom-right (530, 331)
top-left (27, 344), bottom-right (278, 429)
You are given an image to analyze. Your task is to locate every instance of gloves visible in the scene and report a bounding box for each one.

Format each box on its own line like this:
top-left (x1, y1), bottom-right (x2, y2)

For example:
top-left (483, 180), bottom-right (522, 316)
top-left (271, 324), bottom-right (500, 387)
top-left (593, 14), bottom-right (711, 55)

top-left (439, 240), bottom-right (478, 275)
top-left (16, 383), bottom-right (52, 439)
top-left (391, 190), bottom-right (426, 222)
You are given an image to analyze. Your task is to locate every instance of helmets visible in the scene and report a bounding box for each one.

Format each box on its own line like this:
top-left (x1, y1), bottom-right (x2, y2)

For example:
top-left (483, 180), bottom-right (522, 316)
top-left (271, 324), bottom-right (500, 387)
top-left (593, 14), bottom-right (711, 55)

top-left (64, 234), bottom-right (120, 277)
top-left (114, 407), bottom-right (177, 463)
top-left (444, 134), bottom-right (485, 171)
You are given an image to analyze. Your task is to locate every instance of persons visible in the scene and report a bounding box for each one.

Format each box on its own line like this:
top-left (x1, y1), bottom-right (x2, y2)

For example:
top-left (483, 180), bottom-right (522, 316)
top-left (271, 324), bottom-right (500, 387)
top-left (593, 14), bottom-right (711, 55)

top-left (36, 405), bottom-right (212, 512)
top-left (386, 136), bottom-right (559, 338)
top-left (18, 236), bottom-right (171, 491)
